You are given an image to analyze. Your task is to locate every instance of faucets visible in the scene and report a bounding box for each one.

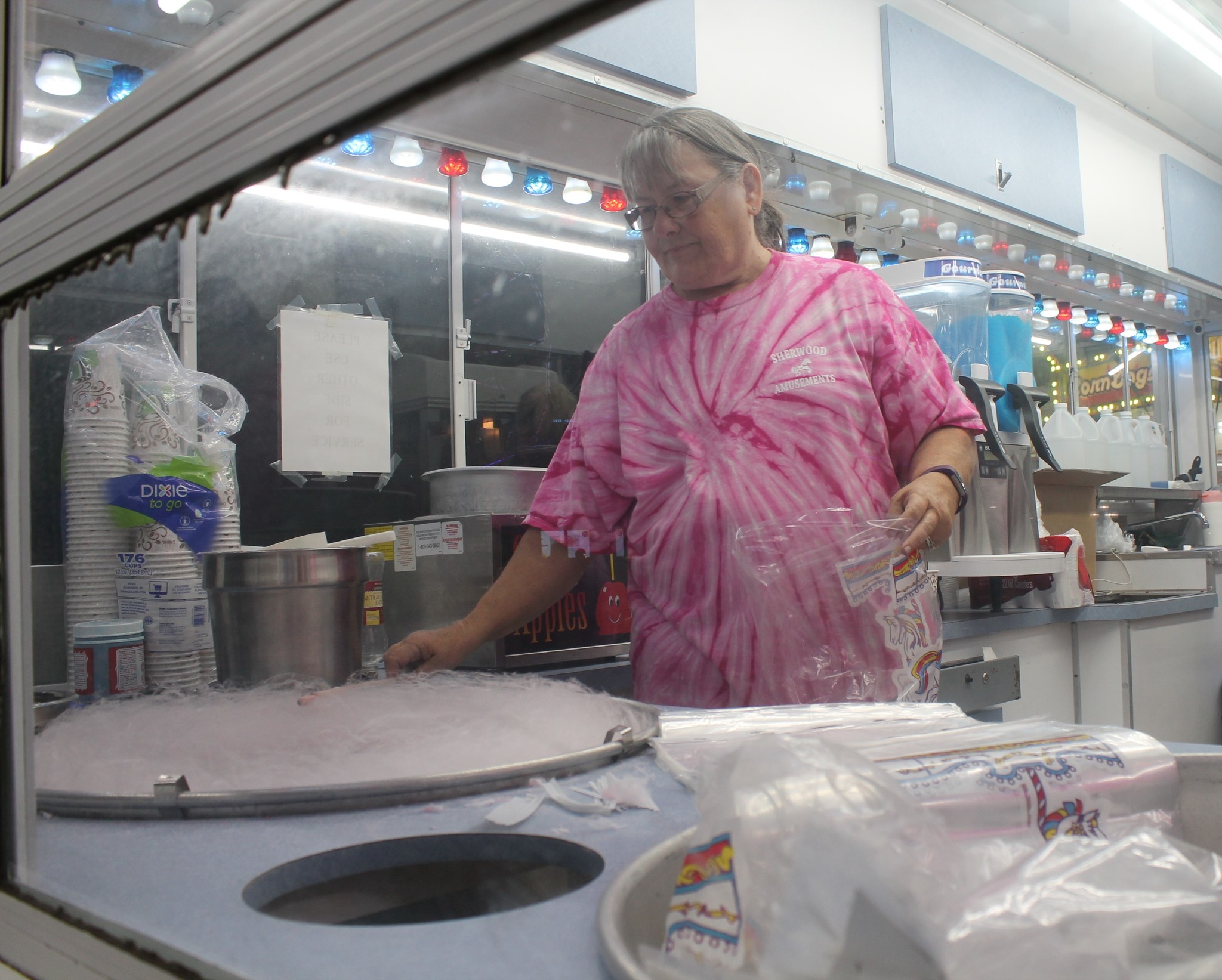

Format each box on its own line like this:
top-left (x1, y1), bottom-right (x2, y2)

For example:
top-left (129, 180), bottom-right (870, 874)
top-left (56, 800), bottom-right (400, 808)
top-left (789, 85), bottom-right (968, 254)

top-left (1122, 511), bottom-right (1210, 533)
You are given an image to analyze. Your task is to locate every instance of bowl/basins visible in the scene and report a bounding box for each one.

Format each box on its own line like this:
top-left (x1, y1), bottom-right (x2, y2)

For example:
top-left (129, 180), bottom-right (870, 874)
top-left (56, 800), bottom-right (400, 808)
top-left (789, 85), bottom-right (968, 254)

top-left (423, 466), bottom-right (546, 517)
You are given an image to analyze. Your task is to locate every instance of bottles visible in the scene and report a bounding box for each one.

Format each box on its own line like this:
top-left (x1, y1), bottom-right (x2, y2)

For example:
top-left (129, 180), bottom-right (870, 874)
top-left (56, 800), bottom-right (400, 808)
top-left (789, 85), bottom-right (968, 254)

top-left (359, 551), bottom-right (388, 671)
top-left (1039, 402), bottom-right (1169, 489)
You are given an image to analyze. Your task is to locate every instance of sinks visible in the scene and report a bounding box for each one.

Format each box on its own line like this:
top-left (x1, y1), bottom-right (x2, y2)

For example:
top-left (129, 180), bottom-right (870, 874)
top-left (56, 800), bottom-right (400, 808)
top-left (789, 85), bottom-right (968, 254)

top-left (1172, 546), bottom-right (1221, 552)
top-left (1093, 593), bottom-right (1188, 604)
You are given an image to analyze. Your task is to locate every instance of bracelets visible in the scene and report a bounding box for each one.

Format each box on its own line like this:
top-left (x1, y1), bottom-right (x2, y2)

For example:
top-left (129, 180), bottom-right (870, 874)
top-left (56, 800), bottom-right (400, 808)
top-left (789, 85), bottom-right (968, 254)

top-left (921, 465), bottom-right (968, 514)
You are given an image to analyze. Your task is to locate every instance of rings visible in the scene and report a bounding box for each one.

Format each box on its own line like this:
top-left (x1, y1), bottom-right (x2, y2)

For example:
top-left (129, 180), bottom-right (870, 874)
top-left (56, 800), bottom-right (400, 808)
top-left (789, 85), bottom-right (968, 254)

top-left (926, 536), bottom-right (936, 552)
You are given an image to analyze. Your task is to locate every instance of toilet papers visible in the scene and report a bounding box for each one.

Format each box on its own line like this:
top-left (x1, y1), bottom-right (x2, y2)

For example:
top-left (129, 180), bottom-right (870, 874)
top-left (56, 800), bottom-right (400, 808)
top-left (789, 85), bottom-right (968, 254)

top-left (969, 534), bottom-right (1083, 610)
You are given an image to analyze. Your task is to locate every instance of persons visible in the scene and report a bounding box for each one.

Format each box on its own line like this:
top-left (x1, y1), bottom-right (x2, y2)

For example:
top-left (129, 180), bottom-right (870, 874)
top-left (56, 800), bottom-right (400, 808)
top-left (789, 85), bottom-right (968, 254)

top-left (492, 381), bottom-right (580, 531)
top-left (383, 108), bottom-right (987, 711)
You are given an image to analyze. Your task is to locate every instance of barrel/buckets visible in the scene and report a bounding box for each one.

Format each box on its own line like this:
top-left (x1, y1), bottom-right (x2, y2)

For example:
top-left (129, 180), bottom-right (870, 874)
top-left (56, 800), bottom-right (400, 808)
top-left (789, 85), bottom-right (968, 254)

top-left (198, 545), bottom-right (375, 689)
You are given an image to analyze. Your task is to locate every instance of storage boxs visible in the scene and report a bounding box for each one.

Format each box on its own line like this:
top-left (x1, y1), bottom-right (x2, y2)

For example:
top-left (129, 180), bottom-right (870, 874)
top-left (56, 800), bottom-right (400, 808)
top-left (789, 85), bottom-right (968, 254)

top-left (1030, 466), bottom-right (1130, 597)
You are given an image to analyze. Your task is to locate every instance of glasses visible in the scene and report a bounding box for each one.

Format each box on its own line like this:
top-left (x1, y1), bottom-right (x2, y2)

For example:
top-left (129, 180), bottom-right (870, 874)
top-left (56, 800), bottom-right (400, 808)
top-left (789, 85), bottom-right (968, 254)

top-left (625, 167), bottom-right (741, 232)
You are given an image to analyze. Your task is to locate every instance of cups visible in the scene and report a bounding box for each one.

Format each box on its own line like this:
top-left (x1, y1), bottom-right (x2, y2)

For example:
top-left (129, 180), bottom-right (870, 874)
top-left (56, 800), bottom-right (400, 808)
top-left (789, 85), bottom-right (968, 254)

top-left (925, 535), bottom-right (1079, 608)
top-left (64, 343), bottom-right (239, 695)
